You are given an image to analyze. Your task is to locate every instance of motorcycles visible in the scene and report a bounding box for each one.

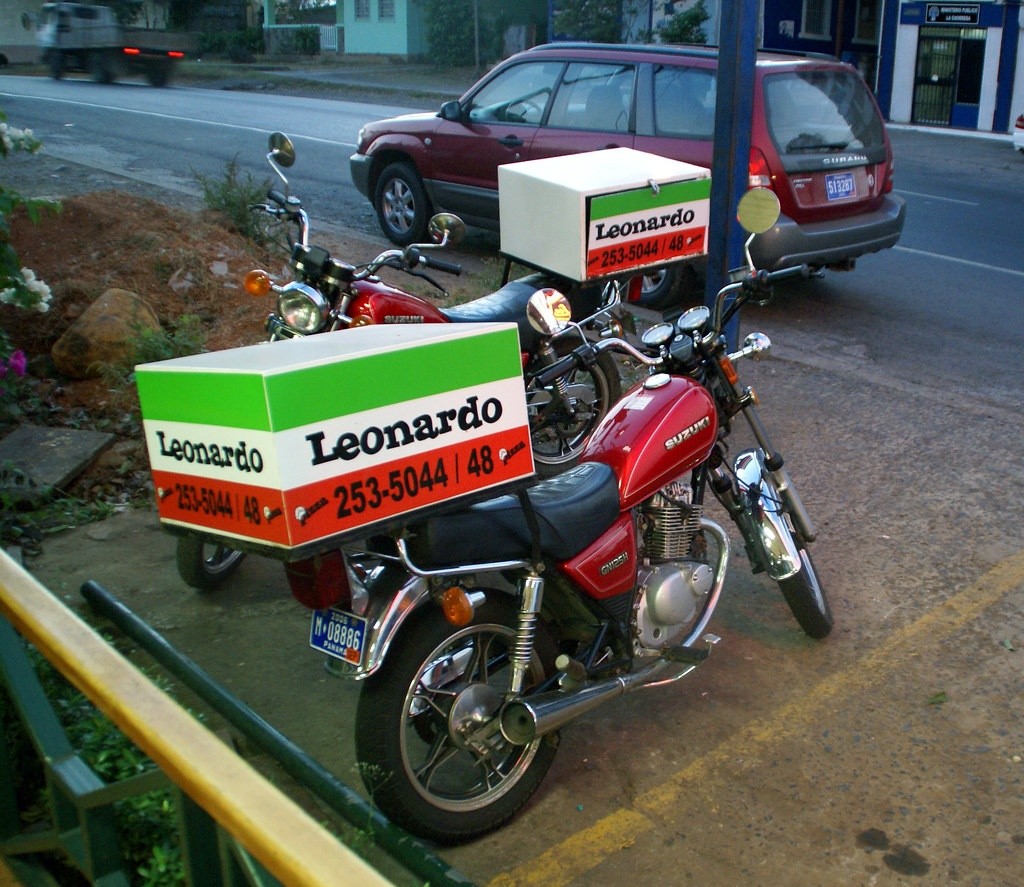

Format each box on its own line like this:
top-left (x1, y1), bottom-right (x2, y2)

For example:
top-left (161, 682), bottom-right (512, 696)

top-left (286, 186), bottom-right (834, 842)
top-left (176, 130), bottom-right (646, 593)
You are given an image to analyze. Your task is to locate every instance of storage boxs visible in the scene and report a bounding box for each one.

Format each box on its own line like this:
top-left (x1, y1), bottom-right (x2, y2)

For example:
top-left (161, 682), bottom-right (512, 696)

top-left (497, 147), bottom-right (713, 280)
top-left (134, 316), bottom-right (540, 557)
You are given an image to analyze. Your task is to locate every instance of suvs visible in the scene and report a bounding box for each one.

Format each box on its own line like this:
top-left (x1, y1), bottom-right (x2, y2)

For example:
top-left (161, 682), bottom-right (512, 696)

top-left (350, 38), bottom-right (909, 310)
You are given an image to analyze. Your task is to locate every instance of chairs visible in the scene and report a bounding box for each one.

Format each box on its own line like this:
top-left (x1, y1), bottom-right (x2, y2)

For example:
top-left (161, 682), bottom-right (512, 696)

top-left (573, 84), bottom-right (623, 128)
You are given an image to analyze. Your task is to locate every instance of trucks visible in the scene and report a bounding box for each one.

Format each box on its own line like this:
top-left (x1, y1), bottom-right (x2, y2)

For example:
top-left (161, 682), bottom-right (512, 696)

top-left (20, 1), bottom-right (208, 89)
